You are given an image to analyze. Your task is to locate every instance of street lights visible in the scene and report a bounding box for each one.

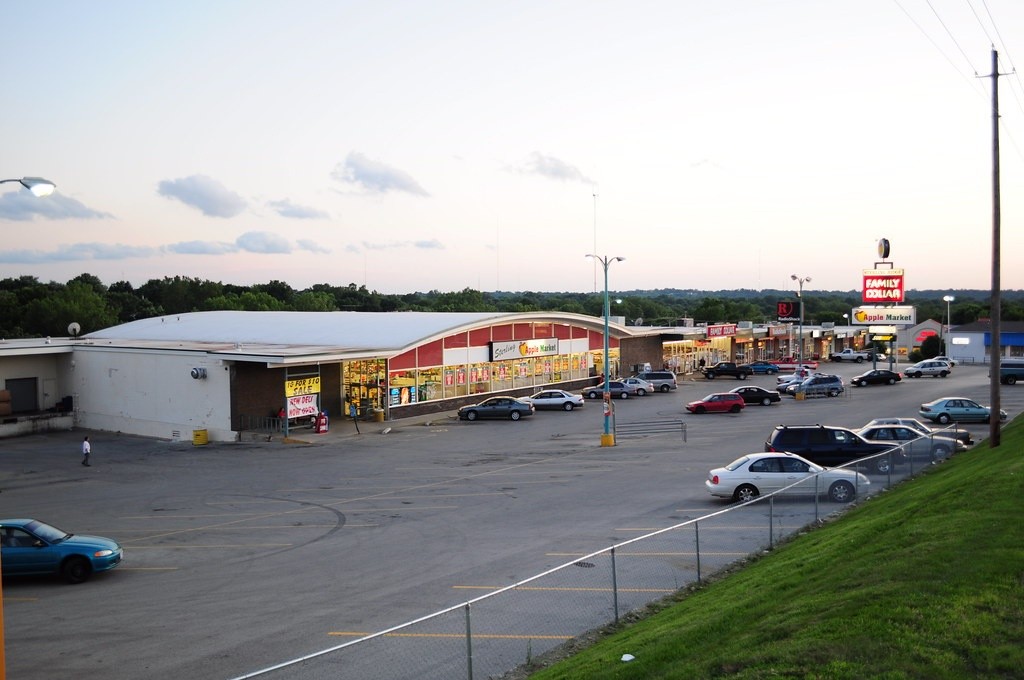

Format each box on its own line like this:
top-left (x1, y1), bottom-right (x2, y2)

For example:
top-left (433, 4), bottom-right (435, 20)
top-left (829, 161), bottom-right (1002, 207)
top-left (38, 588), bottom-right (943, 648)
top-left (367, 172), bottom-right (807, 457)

top-left (791, 275), bottom-right (812, 367)
top-left (943, 296), bottom-right (954, 358)
top-left (843, 314), bottom-right (850, 348)
top-left (585, 254), bottom-right (626, 447)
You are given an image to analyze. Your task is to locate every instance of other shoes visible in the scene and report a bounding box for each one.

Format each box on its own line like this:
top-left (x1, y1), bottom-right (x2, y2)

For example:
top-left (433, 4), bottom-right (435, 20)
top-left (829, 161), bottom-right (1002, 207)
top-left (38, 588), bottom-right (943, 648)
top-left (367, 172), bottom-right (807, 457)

top-left (82, 462), bottom-right (85, 465)
top-left (85, 465), bottom-right (91, 466)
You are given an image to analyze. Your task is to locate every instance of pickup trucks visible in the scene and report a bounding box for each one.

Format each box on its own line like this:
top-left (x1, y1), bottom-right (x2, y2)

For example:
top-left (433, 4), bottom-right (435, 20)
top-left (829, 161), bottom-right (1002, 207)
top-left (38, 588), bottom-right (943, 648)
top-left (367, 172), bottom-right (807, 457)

top-left (768, 356), bottom-right (819, 370)
top-left (702, 362), bottom-right (754, 380)
top-left (828, 348), bottom-right (868, 363)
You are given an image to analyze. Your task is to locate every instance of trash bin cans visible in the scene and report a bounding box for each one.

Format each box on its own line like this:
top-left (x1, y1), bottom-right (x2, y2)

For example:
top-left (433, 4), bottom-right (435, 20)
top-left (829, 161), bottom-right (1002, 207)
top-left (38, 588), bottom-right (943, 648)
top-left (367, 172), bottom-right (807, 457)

top-left (375, 409), bottom-right (384, 422)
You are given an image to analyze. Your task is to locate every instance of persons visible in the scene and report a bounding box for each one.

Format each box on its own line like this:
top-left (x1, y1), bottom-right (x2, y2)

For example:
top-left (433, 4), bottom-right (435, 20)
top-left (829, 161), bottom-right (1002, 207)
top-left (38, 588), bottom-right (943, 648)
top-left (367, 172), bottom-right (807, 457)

top-left (601, 375), bottom-right (605, 383)
top-left (592, 365), bottom-right (596, 376)
top-left (82, 436), bottom-right (90, 466)
top-left (699, 357), bottom-right (705, 366)
top-left (278, 407), bottom-right (286, 428)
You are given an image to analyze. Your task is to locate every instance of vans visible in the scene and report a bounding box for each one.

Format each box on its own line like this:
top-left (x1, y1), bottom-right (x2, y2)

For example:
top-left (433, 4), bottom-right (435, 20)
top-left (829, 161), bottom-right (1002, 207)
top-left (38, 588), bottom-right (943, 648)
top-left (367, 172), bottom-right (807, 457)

top-left (631, 371), bottom-right (678, 393)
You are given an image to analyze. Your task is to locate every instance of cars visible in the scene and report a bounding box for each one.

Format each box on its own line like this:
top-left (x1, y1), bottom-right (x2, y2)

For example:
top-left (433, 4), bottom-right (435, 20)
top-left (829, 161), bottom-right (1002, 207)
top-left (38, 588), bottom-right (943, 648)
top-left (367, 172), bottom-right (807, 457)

top-left (0, 519), bottom-right (124, 583)
top-left (987, 359), bottom-right (1024, 385)
top-left (751, 361), bottom-right (779, 374)
top-left (776, 369), bottom-right (845, 396)
top-left (904, 356), bottom-right (959, 378)
top-left (705, 397), bottom-right (1008, 503)
top-left (581, 381), bottom-right (634, 399)
top-left (517, 390), bottom-right (585, 411)
top-left (617, 378), bottom-right (654, 396)
top-left (685, 386), bottom-right (781, 413)
top-left (850, 369), bottom-right (902, 387)
top-left (457, 396), bottom-right (536, 421)
